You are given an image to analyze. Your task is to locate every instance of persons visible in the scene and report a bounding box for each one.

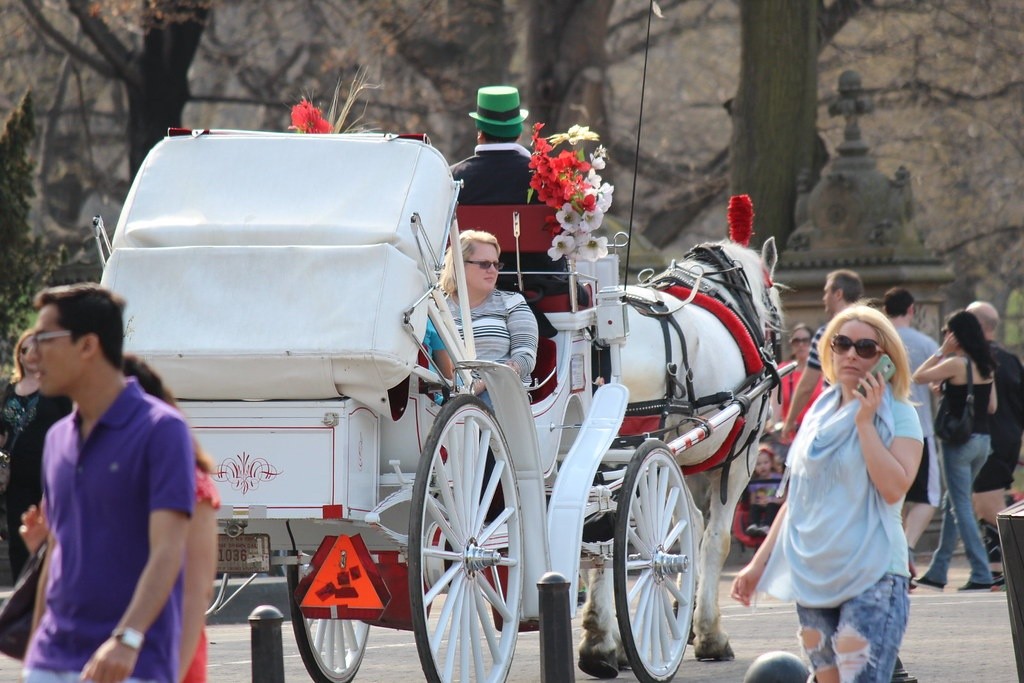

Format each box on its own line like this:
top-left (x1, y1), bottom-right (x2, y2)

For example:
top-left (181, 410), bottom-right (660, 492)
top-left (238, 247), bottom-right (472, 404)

top-left (745, 267), bottom-right (1024, 595)
top-left (731, 305), bottom-right (923, 683)
top-left (417, 230), bottom-right (537, 417)
top-left (0, 281), bottom-right (221, 683)
top-left (447, 85), bottom-right (589, 338)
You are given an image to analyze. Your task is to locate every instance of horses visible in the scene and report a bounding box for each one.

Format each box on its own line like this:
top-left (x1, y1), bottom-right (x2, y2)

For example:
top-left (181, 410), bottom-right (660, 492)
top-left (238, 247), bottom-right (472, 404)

top-left (577, 238), bottom-right (784, 677)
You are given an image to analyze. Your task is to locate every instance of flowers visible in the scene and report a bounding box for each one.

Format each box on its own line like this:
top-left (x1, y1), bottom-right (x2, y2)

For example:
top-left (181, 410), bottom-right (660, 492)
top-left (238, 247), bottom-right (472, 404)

top-left (523, 121), bottom-right (614, 263)
top-left (290, 96), bottom-right (332, 135)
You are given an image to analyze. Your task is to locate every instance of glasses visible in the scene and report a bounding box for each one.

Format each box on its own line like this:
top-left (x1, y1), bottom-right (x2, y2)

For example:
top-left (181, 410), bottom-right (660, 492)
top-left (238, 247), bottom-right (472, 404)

top-left (18, 347), bottom-right (29, 354)
top-left (830, 334), bottom-right (887, 358)
top-left (30, 330), bottom-right (73, 349)
top-left (789, 337), bottom-right (810, 346)
top-left (464, 261), bottom-right (505, 270)
top-left (940, 328), bottom-right (952, 335)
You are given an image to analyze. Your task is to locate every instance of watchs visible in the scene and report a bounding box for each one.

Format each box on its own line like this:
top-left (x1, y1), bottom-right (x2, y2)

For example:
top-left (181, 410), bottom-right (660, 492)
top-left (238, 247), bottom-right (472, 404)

top-left (110, 626), bottom-right (144, 651)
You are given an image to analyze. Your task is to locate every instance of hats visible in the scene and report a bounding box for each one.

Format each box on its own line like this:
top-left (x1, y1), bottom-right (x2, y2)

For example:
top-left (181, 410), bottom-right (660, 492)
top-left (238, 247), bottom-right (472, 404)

top-left (468, 86), bottom-right (529, 138)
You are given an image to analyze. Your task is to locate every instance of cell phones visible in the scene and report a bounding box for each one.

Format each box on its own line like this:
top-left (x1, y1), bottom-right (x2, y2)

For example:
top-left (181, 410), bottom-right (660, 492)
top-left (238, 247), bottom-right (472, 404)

top-left (857, 354), bottom-right (896, 398)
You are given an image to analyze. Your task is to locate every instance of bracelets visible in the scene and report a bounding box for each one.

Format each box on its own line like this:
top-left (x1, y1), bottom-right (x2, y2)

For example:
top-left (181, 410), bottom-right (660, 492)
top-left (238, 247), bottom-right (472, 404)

top-left (935, 350), bottom-right (942, 359)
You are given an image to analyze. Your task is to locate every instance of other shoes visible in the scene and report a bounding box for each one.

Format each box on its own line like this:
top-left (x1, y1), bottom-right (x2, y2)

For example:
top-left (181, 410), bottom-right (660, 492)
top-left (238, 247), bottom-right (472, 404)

top-left (958, 581), bottom-right (994, 592)
top-left (910, 577), bottom-right (944, 591)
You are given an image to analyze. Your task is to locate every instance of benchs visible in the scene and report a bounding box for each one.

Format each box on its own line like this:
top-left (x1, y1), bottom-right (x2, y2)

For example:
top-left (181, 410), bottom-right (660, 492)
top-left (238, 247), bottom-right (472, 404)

top-left (455, 204), bottom-right (596, 329)
top-left (418, 337), bottom-right (559, 477)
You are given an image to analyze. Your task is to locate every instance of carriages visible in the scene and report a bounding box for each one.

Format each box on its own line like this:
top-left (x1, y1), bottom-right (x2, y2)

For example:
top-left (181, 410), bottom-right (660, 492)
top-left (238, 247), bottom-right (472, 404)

top-left (91, 124), bottom-right (799, 683)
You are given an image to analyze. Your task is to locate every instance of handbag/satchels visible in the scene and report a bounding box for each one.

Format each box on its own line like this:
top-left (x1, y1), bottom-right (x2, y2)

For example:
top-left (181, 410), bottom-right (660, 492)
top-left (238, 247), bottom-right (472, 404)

top-left (0, 451), bottom-right (11, 494)
top-left (934, 357), bottom-right (974, 440)
top-left (0, 542), bottom-right (46, 660)
